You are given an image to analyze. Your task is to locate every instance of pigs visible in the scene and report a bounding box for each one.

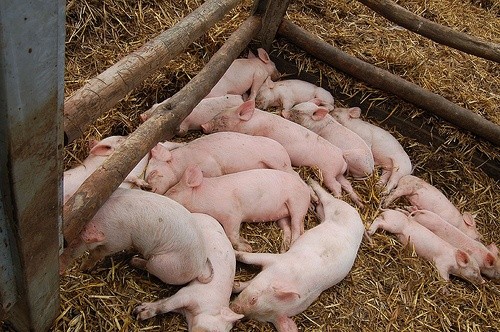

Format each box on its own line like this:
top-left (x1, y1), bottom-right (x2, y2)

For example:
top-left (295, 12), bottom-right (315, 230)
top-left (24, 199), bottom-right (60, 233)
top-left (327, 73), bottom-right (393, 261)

top-left (365, 174), bottom-right (500, 291)
top-left (63, 46), bottom-right (412, 332)
